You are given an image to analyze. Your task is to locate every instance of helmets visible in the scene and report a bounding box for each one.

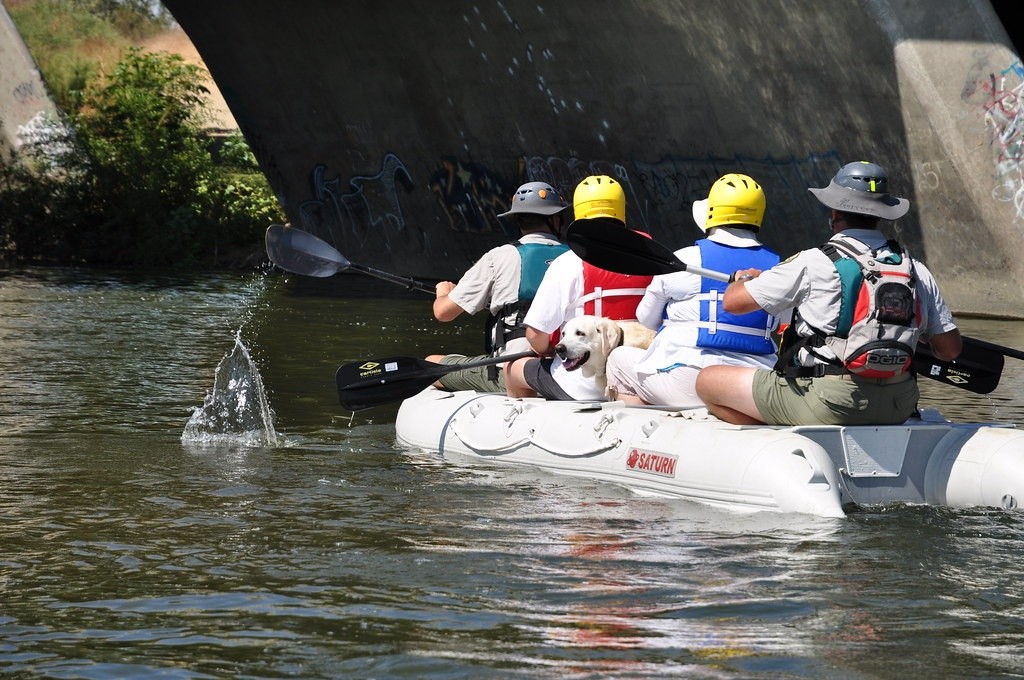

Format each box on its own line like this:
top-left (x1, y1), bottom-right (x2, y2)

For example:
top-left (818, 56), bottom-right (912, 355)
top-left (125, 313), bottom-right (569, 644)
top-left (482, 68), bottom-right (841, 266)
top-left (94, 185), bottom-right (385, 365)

top-left (808, 162), bottom-right (910, 221)
top-left (573, 174), bottom-right (626, 228)
top-left (497, 182), bottom-right (572, 218)
top-left (692, 174), bottom-right (766, 234)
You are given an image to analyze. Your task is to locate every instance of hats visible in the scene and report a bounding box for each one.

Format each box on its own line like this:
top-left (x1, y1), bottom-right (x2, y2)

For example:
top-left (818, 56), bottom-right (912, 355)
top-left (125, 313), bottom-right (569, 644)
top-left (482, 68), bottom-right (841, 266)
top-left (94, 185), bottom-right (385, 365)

top-left (693, 197), bottom-right (763, 248)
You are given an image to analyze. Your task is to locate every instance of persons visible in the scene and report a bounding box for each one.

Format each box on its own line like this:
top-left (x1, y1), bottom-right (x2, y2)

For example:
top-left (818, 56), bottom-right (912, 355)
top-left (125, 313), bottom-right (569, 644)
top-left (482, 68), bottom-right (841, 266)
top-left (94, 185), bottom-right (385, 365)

top-left (423, 181), bottom-right (575, 396)
top-left (603, 172), bottom-right (788, 410)
top-left (694, 160), bottom-right (963, 429)
top-left (503, 174), bottom-right (673, 401)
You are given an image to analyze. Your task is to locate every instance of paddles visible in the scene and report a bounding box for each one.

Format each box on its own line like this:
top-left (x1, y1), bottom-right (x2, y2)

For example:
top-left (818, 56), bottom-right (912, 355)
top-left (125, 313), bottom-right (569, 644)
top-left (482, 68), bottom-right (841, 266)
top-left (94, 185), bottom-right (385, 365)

top-left (334, 349), bottom-right (539, 413)
top-left (562, 217), bottom-right (1024, 362)
top-left (264, 223), bottom-right (438, 295)
top-left (912, 337), bottom-right (1006, 396)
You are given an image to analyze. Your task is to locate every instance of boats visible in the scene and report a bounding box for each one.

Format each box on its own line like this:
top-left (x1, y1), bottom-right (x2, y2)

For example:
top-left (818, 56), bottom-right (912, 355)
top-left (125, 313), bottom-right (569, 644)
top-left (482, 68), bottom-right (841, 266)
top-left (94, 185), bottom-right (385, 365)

top-left (393, 383), bottom-right (1023, 533)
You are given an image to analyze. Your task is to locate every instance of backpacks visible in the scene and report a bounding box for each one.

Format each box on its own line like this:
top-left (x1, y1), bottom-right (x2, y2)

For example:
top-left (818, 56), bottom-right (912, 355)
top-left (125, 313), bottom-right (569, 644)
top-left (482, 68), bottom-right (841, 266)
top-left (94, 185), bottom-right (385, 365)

top-left (808, 244), bottom-right (921, 378)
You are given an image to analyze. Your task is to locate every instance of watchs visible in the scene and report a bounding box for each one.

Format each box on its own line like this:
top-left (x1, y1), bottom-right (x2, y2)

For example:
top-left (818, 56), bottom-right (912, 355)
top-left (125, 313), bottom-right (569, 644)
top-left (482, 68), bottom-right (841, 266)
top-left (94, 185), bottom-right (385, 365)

top-left (738, 273), bottom-right (753, 280)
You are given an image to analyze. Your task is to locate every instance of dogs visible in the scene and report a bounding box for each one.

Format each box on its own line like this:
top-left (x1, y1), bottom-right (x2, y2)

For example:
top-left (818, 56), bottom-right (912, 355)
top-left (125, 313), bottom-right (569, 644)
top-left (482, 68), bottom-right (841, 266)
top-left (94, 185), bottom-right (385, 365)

top-left (554, 315), bottom-right (658, 403)
